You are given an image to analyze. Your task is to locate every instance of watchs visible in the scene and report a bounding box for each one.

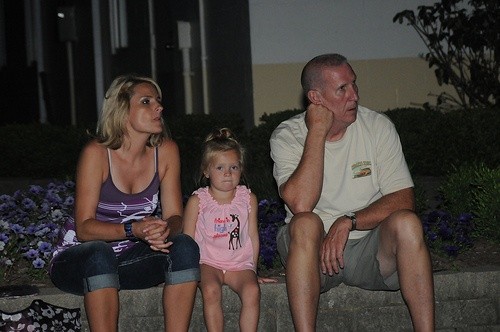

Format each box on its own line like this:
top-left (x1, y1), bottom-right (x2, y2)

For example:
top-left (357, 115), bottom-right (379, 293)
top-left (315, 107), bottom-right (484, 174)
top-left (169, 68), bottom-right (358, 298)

top-left (344, 213), bottom-right (357, 231)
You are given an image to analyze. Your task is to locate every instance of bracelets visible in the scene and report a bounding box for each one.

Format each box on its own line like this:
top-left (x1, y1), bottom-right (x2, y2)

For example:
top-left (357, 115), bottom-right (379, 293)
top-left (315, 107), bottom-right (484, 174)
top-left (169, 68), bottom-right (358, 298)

top-left (124, 220), bottom-right (137, 242)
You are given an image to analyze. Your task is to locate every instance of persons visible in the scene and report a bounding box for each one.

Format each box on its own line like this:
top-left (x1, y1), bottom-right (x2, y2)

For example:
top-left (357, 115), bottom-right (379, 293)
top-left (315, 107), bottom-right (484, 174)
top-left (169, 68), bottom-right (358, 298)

top-left (49, 75), bottom-right (201, 332)
top-left (182, 127), bottom-right (279, 332)
top-left (270, 54), bottom-right (435, 332)
